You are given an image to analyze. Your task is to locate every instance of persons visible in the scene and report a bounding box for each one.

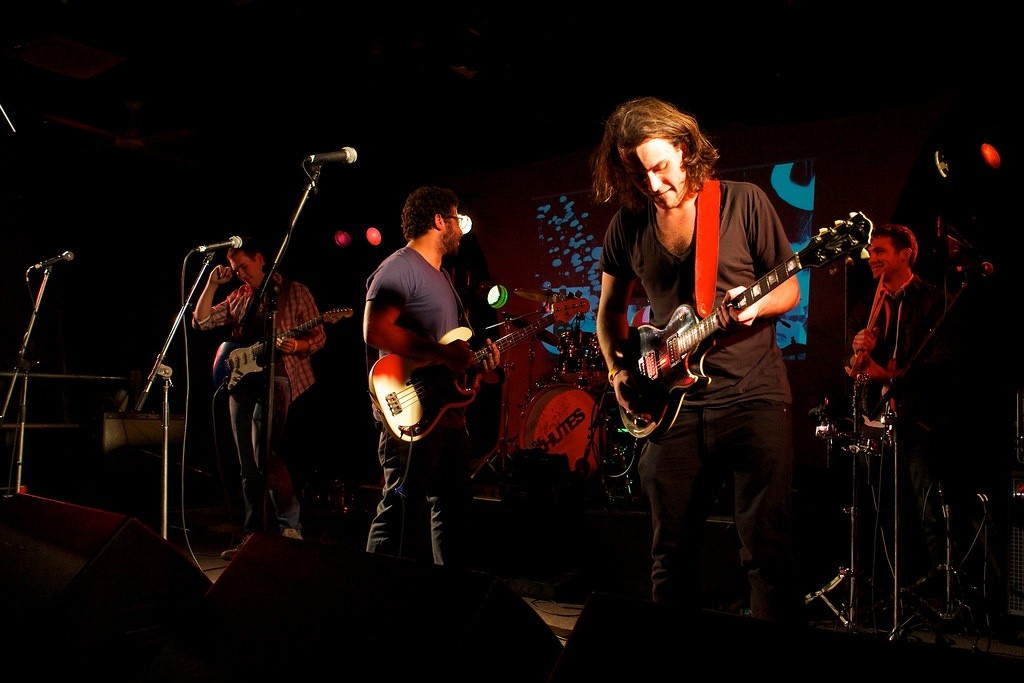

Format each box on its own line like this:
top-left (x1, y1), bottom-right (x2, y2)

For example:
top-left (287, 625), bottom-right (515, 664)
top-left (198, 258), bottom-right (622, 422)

top-left (191, 236), bottom-right (327, 560)
top-left (363, 185), bottom-right (500, 571)
top-left (588, 98), bottom-right (804, 623)
top-left (842, 224), bottom-right (955, 603)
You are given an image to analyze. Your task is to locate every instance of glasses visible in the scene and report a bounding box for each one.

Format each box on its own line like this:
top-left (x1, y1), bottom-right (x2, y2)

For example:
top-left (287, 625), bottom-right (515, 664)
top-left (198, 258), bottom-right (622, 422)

top-left (441, 215), bottom-right (459, 225)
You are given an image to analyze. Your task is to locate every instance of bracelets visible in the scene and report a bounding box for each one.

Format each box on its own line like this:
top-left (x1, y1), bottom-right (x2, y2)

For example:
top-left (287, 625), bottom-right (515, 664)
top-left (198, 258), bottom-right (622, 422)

top-left (608, 366), bottom-right (624, 387)
top-left (293, 339), bottom-right (297, 353)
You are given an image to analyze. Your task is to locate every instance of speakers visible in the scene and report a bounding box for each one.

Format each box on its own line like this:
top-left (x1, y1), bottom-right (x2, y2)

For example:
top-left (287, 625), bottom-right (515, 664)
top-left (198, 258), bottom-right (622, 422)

top-left (0, 467), bottom-right (1024, 683)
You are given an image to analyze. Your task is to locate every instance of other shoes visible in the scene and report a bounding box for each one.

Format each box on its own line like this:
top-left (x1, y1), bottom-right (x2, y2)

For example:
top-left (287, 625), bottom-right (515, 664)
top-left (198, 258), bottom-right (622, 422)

top-left (220, 531), bottom-right (255, 561)
top-left (281, 528), bottom-right (303, 540)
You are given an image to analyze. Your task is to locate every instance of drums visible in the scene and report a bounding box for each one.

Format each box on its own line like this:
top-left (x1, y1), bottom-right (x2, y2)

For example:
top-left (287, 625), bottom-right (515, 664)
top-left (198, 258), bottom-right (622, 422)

top-left (518, 382), bottom-right (640, 498)
top-left (554, 330), bottom-right (609, 388)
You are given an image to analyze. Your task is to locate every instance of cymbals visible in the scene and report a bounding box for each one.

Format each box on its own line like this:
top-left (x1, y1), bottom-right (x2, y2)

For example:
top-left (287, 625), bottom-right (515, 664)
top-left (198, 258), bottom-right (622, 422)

top-left (781, 344), bottom-right (820, 355)
top-left (514, 287), bottom-right (558, 304)
top-left (501, 311), bottom-right (559, 348)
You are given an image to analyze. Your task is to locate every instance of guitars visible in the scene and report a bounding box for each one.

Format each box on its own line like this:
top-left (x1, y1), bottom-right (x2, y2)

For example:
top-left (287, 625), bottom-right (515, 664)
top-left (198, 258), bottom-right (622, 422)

top-left (367, 291), bottom-right (589, 450)
top-left (616, 210), bottom-right (874, 439)
top-left (228, 306), bottom-right (355, 395)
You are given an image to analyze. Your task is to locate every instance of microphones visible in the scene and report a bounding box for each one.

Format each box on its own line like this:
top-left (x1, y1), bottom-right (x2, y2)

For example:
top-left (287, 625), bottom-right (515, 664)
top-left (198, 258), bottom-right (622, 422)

top-left (305, 146), bottom-right (358, 163)
top-left (33, 250), bottom-right (74, 270)
top-left (192, 236), bottom-right (243, 253)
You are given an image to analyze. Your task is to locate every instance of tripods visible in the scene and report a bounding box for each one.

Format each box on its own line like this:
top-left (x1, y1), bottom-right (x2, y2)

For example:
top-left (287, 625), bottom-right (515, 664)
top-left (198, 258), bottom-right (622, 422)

top-left (801, 379), bottom-right (977, 644)
top-left (469, 299), bottom-right (551, 478)
